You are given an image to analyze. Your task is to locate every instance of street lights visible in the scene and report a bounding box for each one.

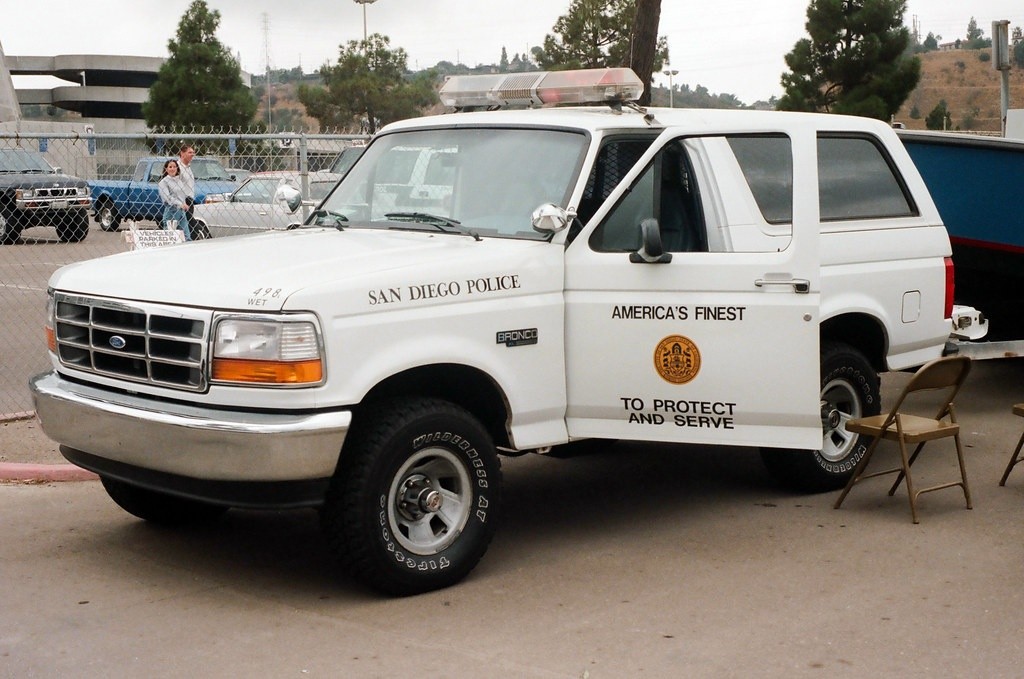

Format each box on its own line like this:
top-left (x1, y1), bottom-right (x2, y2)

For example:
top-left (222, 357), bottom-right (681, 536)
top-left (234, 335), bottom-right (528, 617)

top-left (663, 69), bottom-right (679, 108)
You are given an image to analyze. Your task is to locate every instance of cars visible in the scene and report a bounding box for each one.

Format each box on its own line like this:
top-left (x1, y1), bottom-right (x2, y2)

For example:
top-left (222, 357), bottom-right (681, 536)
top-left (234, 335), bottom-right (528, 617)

top-left (194, 143), bottom-right (458, 241)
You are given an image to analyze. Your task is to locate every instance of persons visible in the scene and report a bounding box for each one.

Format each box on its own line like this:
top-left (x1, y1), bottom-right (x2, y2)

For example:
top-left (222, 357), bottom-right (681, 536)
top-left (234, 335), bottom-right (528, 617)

top-left (177, 144), bottom-right (200, 239)
top-left (157, 159), bottom-right (194, 240)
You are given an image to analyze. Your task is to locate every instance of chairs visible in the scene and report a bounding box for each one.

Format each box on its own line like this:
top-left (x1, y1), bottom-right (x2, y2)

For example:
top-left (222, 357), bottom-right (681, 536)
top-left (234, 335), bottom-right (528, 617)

top-left (834, 353), bottom-right (973, 524)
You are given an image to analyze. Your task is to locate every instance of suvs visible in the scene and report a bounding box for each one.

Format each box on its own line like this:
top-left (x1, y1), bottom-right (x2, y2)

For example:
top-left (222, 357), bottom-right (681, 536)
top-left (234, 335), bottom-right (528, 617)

top-left (25, 67), bottom-right (956, 600)
top-left (1, 147), bottom-right (94, 242)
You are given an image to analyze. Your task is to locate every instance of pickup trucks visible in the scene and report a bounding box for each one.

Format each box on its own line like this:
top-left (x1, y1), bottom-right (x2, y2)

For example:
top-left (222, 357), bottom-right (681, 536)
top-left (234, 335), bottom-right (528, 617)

top-left (84, 156), bottom-right (239, 229)
